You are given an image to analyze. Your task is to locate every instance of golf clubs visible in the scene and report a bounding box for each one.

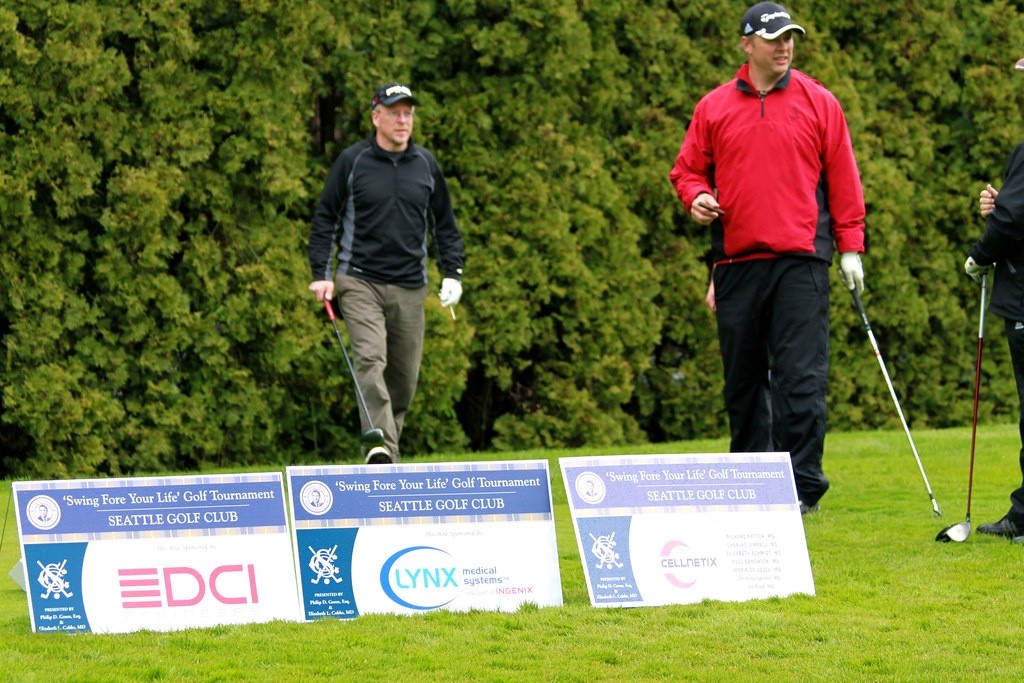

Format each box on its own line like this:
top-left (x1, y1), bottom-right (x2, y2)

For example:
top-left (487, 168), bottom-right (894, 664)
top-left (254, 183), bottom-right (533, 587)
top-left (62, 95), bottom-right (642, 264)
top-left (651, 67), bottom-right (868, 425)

top-left (934, 268), bottom-right (989, 544)
top-left (837, 261), bottom-right (943, 520)
top-left (323, 298), bottom-right (385, 447)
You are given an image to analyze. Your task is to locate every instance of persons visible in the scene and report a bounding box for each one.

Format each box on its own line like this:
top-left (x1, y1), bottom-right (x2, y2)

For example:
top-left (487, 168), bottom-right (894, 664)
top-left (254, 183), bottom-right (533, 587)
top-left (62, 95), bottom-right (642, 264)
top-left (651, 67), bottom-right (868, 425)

top-left (967, 58), bottom-right (1023, 543)
top-left (671, 1), bottom-right (864, 514)
top-left (310, 86), bottom-right (466, 465)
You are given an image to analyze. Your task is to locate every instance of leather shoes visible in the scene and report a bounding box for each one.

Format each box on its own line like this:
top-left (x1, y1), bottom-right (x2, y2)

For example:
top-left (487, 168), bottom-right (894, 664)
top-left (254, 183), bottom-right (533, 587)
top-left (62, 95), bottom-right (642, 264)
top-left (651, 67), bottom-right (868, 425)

top-left (975, 511), bottom-right (1024, 545)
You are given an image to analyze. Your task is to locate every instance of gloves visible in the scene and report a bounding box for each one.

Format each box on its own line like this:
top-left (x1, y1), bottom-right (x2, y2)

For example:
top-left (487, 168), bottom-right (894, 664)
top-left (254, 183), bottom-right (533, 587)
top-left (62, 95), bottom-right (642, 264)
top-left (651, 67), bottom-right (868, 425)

top-left (438, 277), bottom-right (463, 308)
top-left (964, 256), bottom-right (992, 282)
top-left (839, 250), bottom-right (865, 296)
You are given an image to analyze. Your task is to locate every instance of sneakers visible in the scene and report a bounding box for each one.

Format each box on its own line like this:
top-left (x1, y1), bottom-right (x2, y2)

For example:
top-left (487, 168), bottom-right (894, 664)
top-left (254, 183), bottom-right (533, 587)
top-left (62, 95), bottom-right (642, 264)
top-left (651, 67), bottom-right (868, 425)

top-left (365, 443), bottom-right (393, 464)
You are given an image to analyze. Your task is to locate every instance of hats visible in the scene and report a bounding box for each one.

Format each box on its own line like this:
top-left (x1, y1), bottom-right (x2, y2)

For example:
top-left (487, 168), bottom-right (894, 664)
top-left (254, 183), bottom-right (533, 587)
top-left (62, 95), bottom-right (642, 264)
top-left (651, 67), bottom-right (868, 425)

top-left (371, 84), bottom-right (421, 108)
top-left (741, 1), bottom-right (805, 41)
